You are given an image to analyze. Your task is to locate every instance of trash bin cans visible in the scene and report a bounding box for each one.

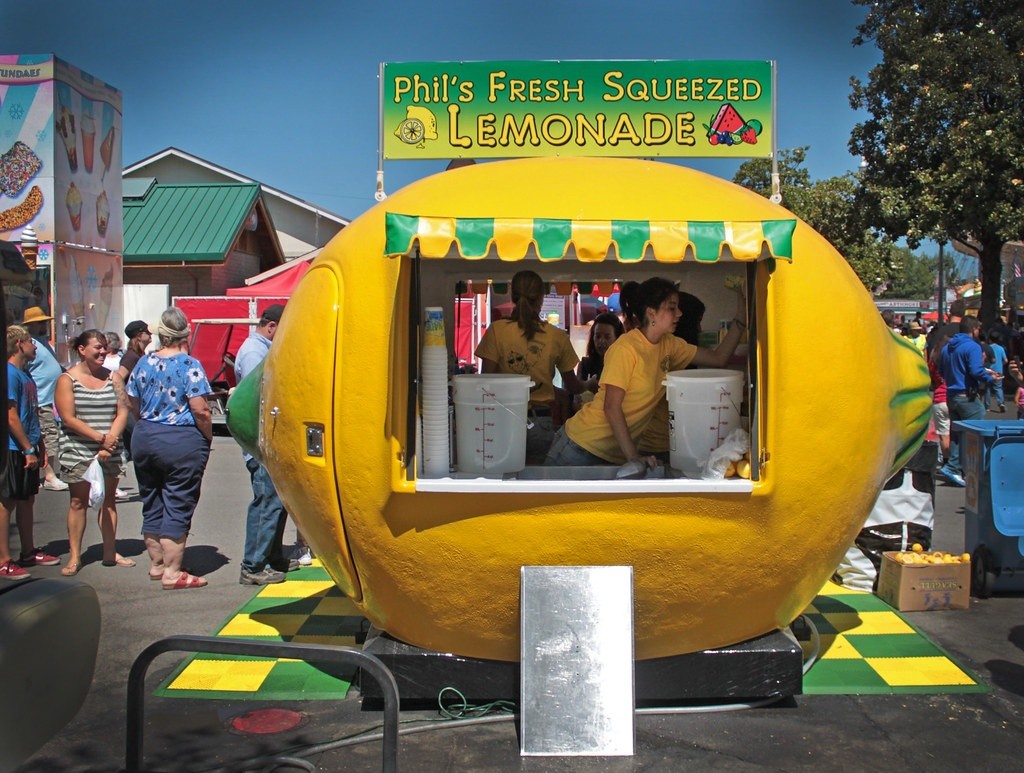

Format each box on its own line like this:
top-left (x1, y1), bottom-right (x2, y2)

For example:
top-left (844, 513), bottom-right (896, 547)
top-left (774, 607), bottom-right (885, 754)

top-left (951, 418), bottom-right (1024, 599)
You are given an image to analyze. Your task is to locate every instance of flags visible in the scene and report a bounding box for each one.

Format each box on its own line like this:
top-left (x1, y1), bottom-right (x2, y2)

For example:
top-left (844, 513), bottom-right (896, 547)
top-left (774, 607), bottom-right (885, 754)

top-left (1012, 252), bottom-right (1021, 279)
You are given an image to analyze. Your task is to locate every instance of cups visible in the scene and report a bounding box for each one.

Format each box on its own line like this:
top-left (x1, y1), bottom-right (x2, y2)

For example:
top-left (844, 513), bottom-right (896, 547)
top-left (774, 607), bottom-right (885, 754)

top-left (415, 390), bottom-right (422, 478)
top-left (579, 394), bottom-right (593, 409)
top-left (422, 306), bottom-right (449, 478)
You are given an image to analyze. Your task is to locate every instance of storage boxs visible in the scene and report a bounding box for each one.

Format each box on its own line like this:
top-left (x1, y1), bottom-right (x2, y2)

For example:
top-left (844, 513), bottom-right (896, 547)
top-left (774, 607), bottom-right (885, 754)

top-left (875, 551), bottom-right (971, 614)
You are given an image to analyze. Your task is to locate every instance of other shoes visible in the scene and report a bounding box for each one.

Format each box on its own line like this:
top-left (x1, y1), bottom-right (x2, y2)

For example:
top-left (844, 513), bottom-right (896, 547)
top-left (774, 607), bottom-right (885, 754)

top-left (939, 465), bottom-right (966, 486)
top-left (114, 489), bottom-right (127, 497)
top-left (985, 407), bottom-right (990, 411)
top-left (43, 479), bottom-right (69, 491)
top-left (1000, 404), bottom-right (1005, 412)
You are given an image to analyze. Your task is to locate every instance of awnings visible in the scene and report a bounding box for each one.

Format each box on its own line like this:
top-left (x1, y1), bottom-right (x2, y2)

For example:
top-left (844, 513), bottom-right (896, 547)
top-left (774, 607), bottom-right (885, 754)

top-left (384, 211), bottom-right (797, 265)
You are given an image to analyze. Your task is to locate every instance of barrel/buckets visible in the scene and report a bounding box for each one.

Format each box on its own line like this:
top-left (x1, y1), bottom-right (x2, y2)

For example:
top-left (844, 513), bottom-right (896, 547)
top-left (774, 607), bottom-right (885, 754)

top-left (451, 373), bottom-right (535, 473)
top-left (661, 368), bottom-right (746, 474)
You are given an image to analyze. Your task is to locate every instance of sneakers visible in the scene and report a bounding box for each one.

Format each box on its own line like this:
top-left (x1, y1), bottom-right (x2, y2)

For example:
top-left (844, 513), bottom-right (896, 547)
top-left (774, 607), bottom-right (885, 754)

top-left (275, 558), bottom-right (300, 571)
top-left (292, 542), bottom-right (312, 565)
top-left (18, 548), bottom-right (60, 565)
top-left (0, 559), bottom-right (30, 580)
top-left (240, 567), bottom-right (286, 585)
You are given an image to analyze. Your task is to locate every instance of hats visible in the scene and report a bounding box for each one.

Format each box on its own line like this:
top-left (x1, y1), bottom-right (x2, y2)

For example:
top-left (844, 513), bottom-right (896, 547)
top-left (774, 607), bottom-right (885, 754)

top-left (125, 320), bottom-right (148, 338)
top-left (908, 322), bottom-right (922, 329)
top-left (147, 318), bottom-right (190, 338)
top-left (21, 307), bottom-right (55, 326)
top-left (262, 304), bottom-right (284, 324)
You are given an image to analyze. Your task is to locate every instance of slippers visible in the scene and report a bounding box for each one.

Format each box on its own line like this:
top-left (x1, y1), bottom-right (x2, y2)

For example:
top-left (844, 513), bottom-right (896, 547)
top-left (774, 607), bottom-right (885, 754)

top-left (103, 558), bottom-right (136, 567)
top-left (151, 574), bottom-right (163, 580)
top-left (163, 571), bottom-right (207, 589)
top-left (62, 562), bottom-right (82, 576)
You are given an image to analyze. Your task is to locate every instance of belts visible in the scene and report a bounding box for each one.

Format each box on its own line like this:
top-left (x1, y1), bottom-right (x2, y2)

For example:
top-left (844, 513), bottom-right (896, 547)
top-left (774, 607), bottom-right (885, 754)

top-left (527, 409), bottom-right (551, 417)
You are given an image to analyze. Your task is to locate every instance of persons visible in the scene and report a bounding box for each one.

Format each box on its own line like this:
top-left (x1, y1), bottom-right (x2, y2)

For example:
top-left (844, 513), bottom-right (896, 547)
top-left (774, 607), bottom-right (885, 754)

top-left (542, 277), bottom-right (747, 465)
top-left (474, 270), bottom-right (598, 465)
top-left (112, 320), bottom-right (152, 496)
top-left (636, 291), bottom-right (705, 462)
top-left (20, 306), bottom-right (69, 490)
top-left (126, 308), bottom-right (212, 590)
top-left (0, 325), bottom-right (60, 581)
top-left (881, 300), bottom-right (1024, 487)
top-left (233, 304), bottom-right (314, 587)
top-left (54, 329), bottom-right (138, 577)
top-left (576, 312), bottom-right (626, 394)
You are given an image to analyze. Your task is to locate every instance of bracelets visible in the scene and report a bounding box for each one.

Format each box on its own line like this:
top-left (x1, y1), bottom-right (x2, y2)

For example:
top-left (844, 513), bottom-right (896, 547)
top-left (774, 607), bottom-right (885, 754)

top-left (22, 446), bottom-right (35, 455)
top-left (100, 434), bottom-right (106, 445)
top-left (733, 318), bottom-right (746, 328)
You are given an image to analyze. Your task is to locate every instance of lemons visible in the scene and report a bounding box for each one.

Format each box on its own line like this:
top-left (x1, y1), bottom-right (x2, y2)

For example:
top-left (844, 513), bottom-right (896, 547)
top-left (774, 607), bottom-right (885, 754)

top-left (722, 452), bottom-right (751, 479)
top-left (894, 543), bottom-right (971, 564)
